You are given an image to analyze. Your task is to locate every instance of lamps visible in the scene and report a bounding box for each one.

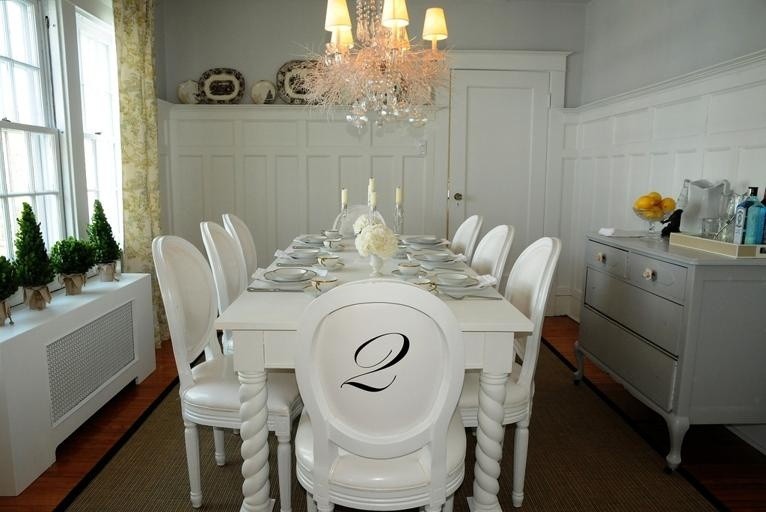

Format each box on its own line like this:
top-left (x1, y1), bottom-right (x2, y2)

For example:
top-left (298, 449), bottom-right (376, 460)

top-left (295, 0), bottom-right (455, 134)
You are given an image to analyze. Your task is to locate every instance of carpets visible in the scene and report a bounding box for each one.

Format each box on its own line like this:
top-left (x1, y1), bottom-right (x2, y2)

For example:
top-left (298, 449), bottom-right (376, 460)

top-left (51, 329), bottom-right (727, 512)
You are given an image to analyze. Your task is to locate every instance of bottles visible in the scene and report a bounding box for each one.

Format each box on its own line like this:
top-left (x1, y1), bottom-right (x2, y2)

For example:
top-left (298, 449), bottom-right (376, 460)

top-left (735, 184), bottom-right (763, 246)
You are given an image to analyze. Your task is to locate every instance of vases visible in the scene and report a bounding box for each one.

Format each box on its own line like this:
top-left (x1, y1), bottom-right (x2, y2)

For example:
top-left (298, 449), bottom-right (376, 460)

top-left (370, 252), bottom-right (384, 277)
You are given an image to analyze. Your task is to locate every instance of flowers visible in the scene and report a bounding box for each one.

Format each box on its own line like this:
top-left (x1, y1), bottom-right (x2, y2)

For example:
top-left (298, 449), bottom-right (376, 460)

top-left (355, 223), bottom-right (397, 259)
top-left (353, 211), bottom-right (385, 235)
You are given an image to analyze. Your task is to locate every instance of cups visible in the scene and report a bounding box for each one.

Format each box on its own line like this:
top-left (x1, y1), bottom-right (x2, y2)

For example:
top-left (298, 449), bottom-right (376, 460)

top-left (701, 217), bottom-right (719, 239)
top-left (317, 256), bottom-right (340, 268)
top-left (310, 275), bottom-right (339, 295)
top-left (325, 230), bottom-right (339, 238)
top-left (323, 239), bottom-right (341, 249)
top-left (398, 243), bottom-right (407, 252)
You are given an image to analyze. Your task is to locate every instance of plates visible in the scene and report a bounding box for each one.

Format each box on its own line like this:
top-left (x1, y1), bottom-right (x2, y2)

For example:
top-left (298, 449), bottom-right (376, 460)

top-left (312, 261), bottom-right (346, 270)
top-left (319, 245), bottom-right (345, 250)
top-left (631, 205), bottom-right (675, 243)
top-left (391, 269), bottom-right (428, 281)
top-left (287, 252), bottom-right (328, 262)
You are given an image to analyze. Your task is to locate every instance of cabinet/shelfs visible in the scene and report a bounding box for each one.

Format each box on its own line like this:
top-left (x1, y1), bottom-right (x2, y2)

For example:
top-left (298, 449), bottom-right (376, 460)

top-left (571, 232), bottom-right (766, 471)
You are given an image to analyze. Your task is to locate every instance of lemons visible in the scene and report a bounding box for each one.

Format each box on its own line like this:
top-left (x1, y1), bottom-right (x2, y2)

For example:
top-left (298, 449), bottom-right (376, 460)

top-left (660, 198), bottom-right (677, 210)
top-left (645, 210), bottom-right (655, 220)
top-left (652, 206), bottom-right (664, 217)
top-left (648, 192), bottom-right (661, 205)
top-left (636, 196), bottom-right (654, 210)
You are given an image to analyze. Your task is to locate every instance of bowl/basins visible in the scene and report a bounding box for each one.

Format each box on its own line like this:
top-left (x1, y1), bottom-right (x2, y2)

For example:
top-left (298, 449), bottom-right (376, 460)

top-left (273, 267), bottom-right (307, 280)
top-left (262, 270), bottom-right (318, 285)
top-left (396, 262), bottom-right (422, 275)
top-left (412, 255), bottom-right (456, 267)
top-left (402, 237), bottom-right (447, 251)
top-left (433, 277), bottom-right (481, 297)
top-left (293, 246), bottom-right (320, 258)
top-left (434, 271), bottom-right (469, 284)
top-left (422, 251), bottom-right (450, 261)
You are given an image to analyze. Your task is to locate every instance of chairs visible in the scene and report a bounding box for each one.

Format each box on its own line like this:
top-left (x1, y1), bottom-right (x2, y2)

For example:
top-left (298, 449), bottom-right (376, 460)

top-left (460, 236), bottom-right (564, 507)
top-left (468, 223), bottom-right (516, 297)
top-left (222, 212), bottom-right (259, 284)
top-left (294, 278), bottom-right (466, 511)
top-left (332, 204), bottom-right (388, 235)
top-left (199, 217), bottom-right (249, 354)
top-left (151, 235), bottom-right (302, 511)
top-left (451, 216), bottom-right (482, 264)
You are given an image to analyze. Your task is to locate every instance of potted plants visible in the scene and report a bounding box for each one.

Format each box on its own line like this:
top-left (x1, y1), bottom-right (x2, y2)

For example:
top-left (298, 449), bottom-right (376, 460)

top-left (87, 200), bottom-right (123, 283)
top-left (52, 238), bottom-right (95, 296)
top-left (0, 257), bottom-right (19, 329)
top-left (12, 201), bottom-right (55, 310)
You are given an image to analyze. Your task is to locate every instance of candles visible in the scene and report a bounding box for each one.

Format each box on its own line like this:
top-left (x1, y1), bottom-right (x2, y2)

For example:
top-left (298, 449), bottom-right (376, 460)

top-left (342, 188), bottom-right (348, 204)
top-left (371, 190), bottom-right (377, 207)
top-left (396, 185), bottom-right (402, 204)
top-left (369, 177), bottom-right (375, 192)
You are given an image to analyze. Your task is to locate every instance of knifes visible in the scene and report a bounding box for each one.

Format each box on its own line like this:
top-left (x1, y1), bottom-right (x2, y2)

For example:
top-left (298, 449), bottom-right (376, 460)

top-left (247, 287), bottom-right (304, 292)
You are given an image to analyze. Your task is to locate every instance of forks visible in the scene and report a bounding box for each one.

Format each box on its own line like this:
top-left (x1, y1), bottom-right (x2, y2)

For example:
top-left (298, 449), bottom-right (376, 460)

top-left (446, 293), bottom-right (504, 301)
